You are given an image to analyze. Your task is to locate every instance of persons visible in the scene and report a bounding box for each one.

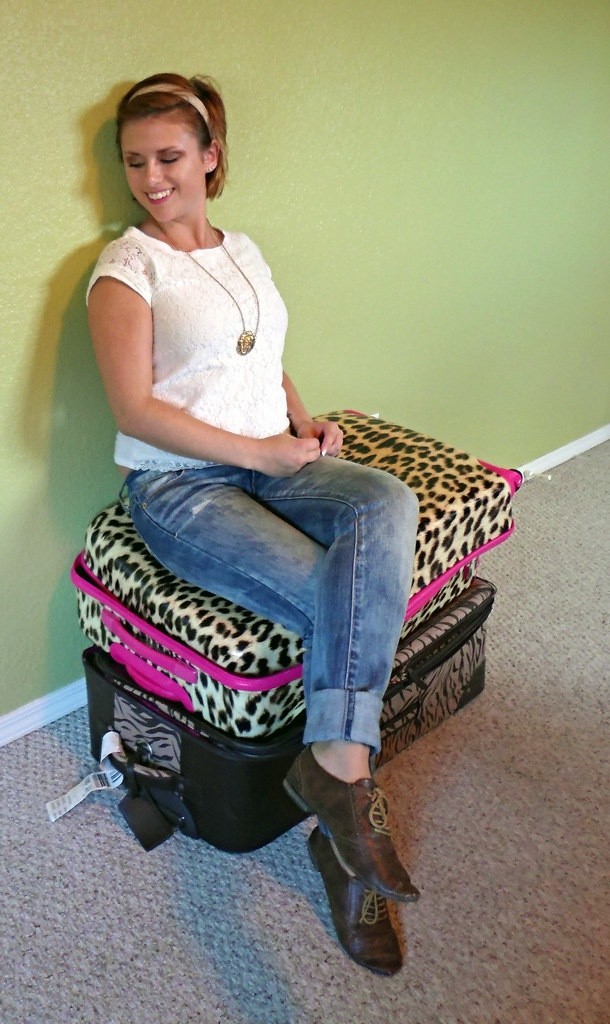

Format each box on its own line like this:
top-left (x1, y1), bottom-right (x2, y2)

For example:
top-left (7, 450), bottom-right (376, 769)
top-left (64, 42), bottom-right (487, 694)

top-left (82, 73), bottom-right (420, 978)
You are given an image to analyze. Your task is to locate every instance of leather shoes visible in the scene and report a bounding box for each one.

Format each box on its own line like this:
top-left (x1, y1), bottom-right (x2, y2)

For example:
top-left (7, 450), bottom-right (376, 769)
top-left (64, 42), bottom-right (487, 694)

top-left (282, 744), bottom-right (421, 903)
top-left (307, 825), bottom-right (402, 976)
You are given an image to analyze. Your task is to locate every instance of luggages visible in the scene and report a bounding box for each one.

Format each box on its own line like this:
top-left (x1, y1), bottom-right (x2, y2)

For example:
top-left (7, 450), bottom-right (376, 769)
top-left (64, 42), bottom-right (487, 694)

top-left (71, 409), bottom-right (523, 740)
top-left (81, 576), bottom-right (498, 855)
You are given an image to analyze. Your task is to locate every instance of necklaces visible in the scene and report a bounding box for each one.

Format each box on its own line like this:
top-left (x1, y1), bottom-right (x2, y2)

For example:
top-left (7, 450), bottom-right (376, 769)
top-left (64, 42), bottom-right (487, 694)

top-left (155, 218), bottom-right (260, 355)
